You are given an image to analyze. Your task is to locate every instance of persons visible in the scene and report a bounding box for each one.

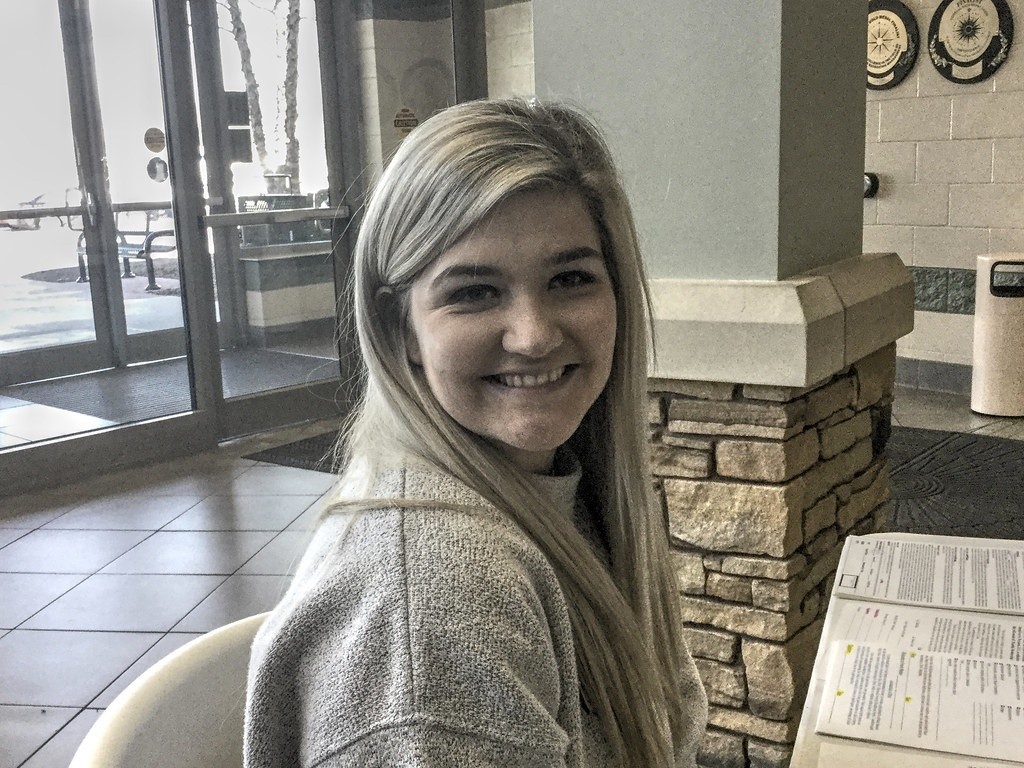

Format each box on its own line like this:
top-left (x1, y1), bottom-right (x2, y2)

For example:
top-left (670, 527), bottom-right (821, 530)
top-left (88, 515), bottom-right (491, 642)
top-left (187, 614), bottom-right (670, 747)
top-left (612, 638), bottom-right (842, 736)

top-left (243, 99), bottom-right (710, 768)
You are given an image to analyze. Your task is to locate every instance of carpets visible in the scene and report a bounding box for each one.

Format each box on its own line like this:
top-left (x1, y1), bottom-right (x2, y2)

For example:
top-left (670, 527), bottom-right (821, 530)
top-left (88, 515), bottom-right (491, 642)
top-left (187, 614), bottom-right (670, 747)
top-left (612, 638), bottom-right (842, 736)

top-left (0, 344), bottom-right (340, 425)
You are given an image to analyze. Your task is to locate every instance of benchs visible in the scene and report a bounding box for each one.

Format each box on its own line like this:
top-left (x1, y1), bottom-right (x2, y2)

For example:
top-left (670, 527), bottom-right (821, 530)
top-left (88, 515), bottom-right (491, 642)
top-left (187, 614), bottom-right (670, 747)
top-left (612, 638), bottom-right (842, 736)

top-left (76, 211), bottom-right (179, 291)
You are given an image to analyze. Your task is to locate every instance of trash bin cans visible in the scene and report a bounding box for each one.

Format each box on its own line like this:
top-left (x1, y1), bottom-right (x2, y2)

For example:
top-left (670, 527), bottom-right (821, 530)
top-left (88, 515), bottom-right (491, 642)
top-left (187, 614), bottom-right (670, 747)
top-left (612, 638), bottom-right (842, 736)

top-left (970, 251), bottom-right (1024, 419)
top-left (238, 196), bottom-right (307, 245)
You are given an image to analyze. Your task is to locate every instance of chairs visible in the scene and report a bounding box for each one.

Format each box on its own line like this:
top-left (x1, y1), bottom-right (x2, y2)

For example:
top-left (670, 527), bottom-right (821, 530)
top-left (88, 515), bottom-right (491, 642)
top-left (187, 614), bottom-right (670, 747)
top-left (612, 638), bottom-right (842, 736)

top-left (70, 607), bottom-right (271, 768)
